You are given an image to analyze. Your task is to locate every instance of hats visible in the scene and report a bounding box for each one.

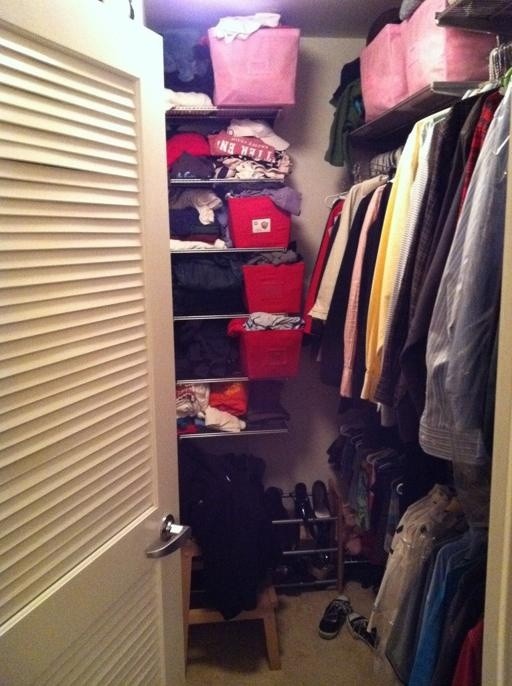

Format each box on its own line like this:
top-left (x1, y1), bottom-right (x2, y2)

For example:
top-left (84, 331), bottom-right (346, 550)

top-left (227, 119), bottom-right (292, 152)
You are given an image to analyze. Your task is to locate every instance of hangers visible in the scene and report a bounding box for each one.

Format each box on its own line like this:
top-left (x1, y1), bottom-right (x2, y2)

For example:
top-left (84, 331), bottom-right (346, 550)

top-left (462, 40), bottom-right (512, 100)
top-left (340, 422), bottom-right (490, 538)
top-left (323, 145), bottom-right (407, 210)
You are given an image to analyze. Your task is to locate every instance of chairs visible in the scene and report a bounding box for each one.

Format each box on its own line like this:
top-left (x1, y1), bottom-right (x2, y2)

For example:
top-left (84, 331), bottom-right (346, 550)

top-left (182, 539), bottom-right (282, 675)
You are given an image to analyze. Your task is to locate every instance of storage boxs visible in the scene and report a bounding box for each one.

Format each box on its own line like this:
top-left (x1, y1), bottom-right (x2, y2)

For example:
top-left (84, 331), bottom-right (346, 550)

top-left (208, 24), bottom-right (302, 108)
top-left (239, 329), bottom-right (303, 380)
top-left (241, 262), bottom-right (304, 314)
top-left (226, 196), bottom-right (291, 248)
top-left (359, 22), bottom-right (408, 124)
top-left (399, 0), bottom-right (499, 97)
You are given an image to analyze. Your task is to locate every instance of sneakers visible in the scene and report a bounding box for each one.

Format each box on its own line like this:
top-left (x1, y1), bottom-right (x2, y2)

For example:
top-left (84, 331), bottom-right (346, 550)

top-left (319, 594), bottom-right (350, 639)
top-left (347, 611), bottom-right (381, 652)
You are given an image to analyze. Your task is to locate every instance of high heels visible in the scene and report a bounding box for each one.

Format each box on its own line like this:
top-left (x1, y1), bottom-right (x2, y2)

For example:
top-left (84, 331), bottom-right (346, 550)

top-left (310, 479), bottom-right (330, 518)
top-left (294, 482), bottom-right (311, 523)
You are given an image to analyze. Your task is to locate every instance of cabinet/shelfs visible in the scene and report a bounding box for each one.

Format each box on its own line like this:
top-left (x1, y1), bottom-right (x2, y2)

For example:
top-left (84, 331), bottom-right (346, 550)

top-left (347, 1), bottom-right (511, 152)
top-left (165, 106), bottom-right (291, 443)
top-left (269, 488), bottom-right (343, 592)
